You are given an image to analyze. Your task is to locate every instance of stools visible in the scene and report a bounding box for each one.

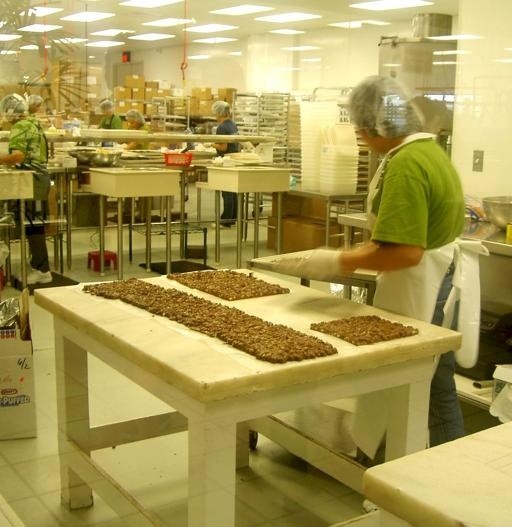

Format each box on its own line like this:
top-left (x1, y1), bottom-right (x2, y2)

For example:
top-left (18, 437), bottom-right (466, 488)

top-left (87, 250), bottom-right (117, 271)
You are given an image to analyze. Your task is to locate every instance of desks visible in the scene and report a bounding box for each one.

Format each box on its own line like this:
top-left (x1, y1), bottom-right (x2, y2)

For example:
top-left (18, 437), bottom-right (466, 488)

top-left (194, 164), bottom-right (291, 269)
top-left (89, 168), bottom-right (183, 280)
top-left (361, 420), bottom-right (511, 527)
top-left (32, 268), bottom-right (463, 527)
top-left (0, 167), bottom-right (34, 292)
top-left (280, 190), bottom-right (367, 249)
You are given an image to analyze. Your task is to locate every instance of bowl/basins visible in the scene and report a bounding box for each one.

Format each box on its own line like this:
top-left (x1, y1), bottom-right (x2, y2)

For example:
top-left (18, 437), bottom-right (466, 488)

top-left (87, 151), bottom-right (123, 168)
top-left (483, 196), bottom-right (512, 230)
top-left (69, 149), bottom-right (96, 164)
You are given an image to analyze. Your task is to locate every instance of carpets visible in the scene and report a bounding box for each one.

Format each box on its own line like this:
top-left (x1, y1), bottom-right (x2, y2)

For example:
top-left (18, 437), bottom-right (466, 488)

top-left (132, 223), bottom-right (204, 237)
top-left (11, 271), bottom-right (80, 296)
top-left (138, 260), bottom-right (215, 275)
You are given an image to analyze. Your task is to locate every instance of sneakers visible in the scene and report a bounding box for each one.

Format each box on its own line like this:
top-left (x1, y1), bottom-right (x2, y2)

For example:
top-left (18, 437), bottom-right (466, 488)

top-left (18, 270), bottom-right (53, 284)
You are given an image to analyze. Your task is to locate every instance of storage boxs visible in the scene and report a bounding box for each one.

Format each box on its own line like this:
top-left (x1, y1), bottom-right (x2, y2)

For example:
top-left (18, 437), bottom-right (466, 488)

top-left (114, 73), bottom-right (237, 118)
top-left (0, 318), bottom-right (38, 441)
top-left (267, 214), bottom-right (341, 254)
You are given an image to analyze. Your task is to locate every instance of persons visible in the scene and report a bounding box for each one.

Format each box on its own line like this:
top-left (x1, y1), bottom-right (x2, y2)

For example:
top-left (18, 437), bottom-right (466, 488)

top-left (202, 99), bottom-right (240, 229)
top-left (0, 93), bottom-right (54, 285)
top-left (96, 100), bottom-right (123, 147)
top-left (24, 95), bottom-right (44, 116)
top-left (305, 74), bottom-right (468, 467)
top-left (114, 110), bottom-right (151, 221)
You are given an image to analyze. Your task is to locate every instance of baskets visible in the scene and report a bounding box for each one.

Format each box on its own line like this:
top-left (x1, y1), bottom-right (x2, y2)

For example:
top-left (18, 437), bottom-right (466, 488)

top-left (163, 153), bottom-right (193, 166)
